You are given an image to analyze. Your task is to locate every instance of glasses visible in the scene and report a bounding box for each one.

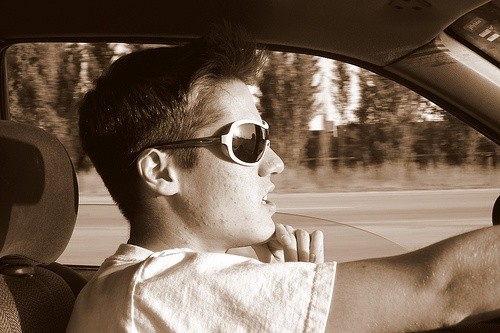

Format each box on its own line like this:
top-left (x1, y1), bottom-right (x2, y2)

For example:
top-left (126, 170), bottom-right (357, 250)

top-left (127, 119), bottom-right (271, 166)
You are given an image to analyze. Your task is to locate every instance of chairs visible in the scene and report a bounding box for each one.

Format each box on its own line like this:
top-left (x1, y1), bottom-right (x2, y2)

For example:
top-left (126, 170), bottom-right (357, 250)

top-left (0, 121), bottom-right (87, 333)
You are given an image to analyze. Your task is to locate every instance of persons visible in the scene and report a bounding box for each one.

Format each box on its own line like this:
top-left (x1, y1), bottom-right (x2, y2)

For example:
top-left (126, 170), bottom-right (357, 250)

top-left (66, 21), bottom-right (500, 333)
